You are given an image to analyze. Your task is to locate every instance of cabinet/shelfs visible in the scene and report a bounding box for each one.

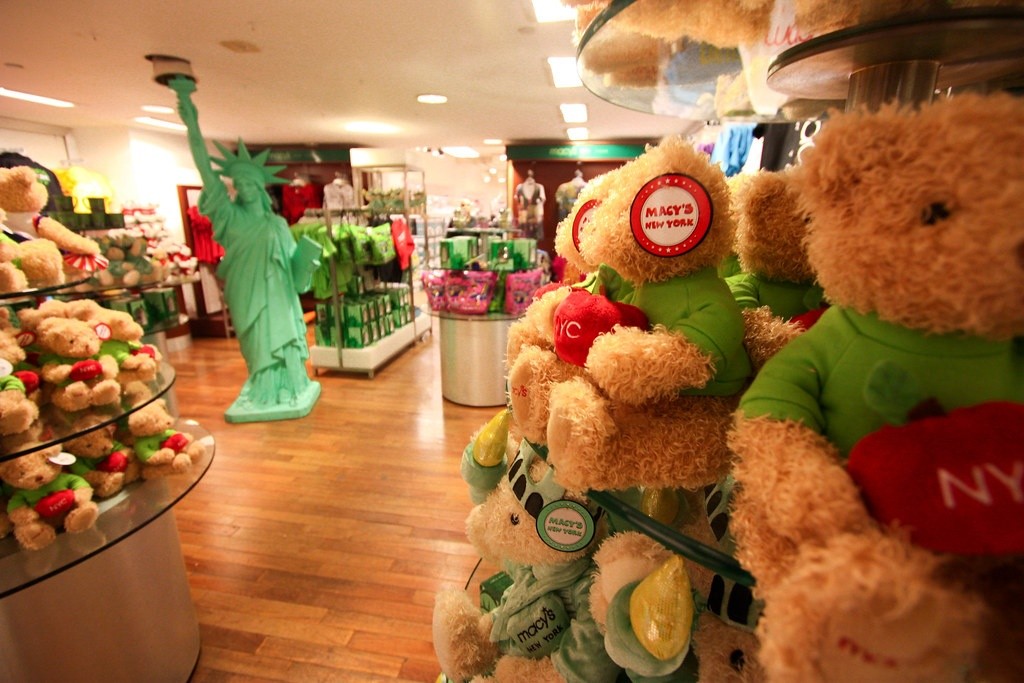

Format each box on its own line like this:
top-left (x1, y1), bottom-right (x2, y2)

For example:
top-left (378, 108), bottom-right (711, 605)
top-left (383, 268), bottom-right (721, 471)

top-left (304, 162), bottom-right (432, 370)
top-left (425, 228), bottom-right (550, 408)
top-left (1, 272), bottom-right (216, 683)
top-left (503, 1), bottom-right (1024, 589)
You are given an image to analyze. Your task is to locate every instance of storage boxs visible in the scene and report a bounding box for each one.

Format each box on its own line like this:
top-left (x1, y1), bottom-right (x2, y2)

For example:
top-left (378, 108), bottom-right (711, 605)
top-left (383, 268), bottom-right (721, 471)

top-left (440, 237), bottom-right (539, 272)
top-left (46, 197), bottom-right (179, 330)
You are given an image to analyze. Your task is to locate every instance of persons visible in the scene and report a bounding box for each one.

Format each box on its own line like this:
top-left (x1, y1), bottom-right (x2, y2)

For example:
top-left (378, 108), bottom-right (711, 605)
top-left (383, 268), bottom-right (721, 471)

top-left (177, 98), bottom-right (321, 410)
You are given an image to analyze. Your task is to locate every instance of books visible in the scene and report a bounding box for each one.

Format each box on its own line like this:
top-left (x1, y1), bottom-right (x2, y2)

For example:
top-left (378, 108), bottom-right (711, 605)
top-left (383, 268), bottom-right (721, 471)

top-left (291, 235), bottom-right (322, 280)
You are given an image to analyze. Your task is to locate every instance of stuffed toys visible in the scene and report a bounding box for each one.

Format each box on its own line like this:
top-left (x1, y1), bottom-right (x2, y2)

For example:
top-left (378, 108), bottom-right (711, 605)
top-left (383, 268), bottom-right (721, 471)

top-left (1, 166), bottom-right (205, 550)
top-left (430, 0), bottom-right (1024, 683)
